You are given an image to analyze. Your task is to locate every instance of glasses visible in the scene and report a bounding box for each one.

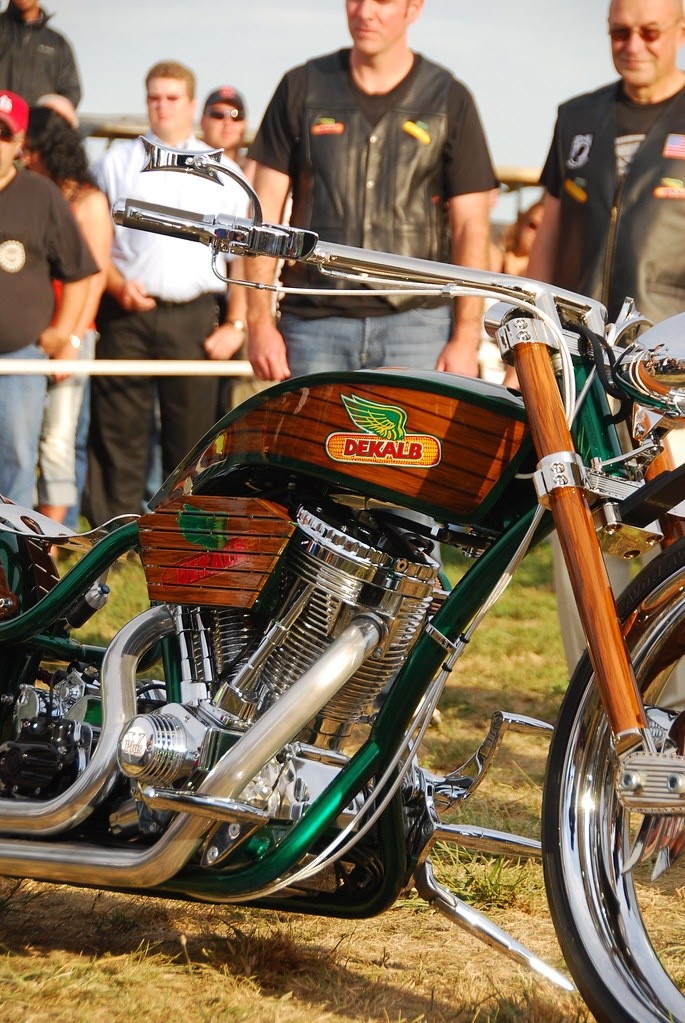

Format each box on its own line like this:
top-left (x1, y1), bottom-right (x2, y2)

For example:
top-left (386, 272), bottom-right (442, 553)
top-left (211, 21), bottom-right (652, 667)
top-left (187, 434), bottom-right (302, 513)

top-left (209, 110), bottom-right (244, 122)
top-left (149, 94), bottom-right (189, 101)
top-left (608, 17), bottom-right (681, 41)
top-left (0, 128), bottom-right (16, 142)
top-left (525, 218), bottom-right (538, 230)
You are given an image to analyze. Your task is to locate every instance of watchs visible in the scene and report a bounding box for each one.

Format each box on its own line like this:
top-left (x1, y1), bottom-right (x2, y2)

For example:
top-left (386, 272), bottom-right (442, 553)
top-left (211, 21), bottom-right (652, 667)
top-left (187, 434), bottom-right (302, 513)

top-left (67, 335), bottom-right (82, 351)
top-left (226, 319), bottom-right (247, 335)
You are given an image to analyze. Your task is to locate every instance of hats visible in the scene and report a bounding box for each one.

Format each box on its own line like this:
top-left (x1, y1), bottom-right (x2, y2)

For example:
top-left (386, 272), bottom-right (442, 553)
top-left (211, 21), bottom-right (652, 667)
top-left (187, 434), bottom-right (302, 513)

top-left (206, 88), bottom-right (243, 111)
top-left (0, 89), bottom-right (30, 134)
top-left (37, 94), bottom-right (79, 130)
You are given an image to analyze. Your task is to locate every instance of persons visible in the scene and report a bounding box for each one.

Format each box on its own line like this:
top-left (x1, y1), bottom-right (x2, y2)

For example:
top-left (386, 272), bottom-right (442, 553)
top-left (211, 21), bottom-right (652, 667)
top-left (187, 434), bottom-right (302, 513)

top-left (239, 1), bottom-right (502, 385)
top-left (520, 0), bottom-right (685, 722)
top-left (478, 165), bottom-right (543, 390)
top-left (1, 0), bottom-right (118, 561)
top-left (81, 62), bottom-right (251, 537)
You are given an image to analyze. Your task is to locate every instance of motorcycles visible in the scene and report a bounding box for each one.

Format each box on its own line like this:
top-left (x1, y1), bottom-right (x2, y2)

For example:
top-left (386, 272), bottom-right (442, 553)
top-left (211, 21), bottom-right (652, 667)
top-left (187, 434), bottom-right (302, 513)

top-left (1, 136), bottom-right (684, 1023)
